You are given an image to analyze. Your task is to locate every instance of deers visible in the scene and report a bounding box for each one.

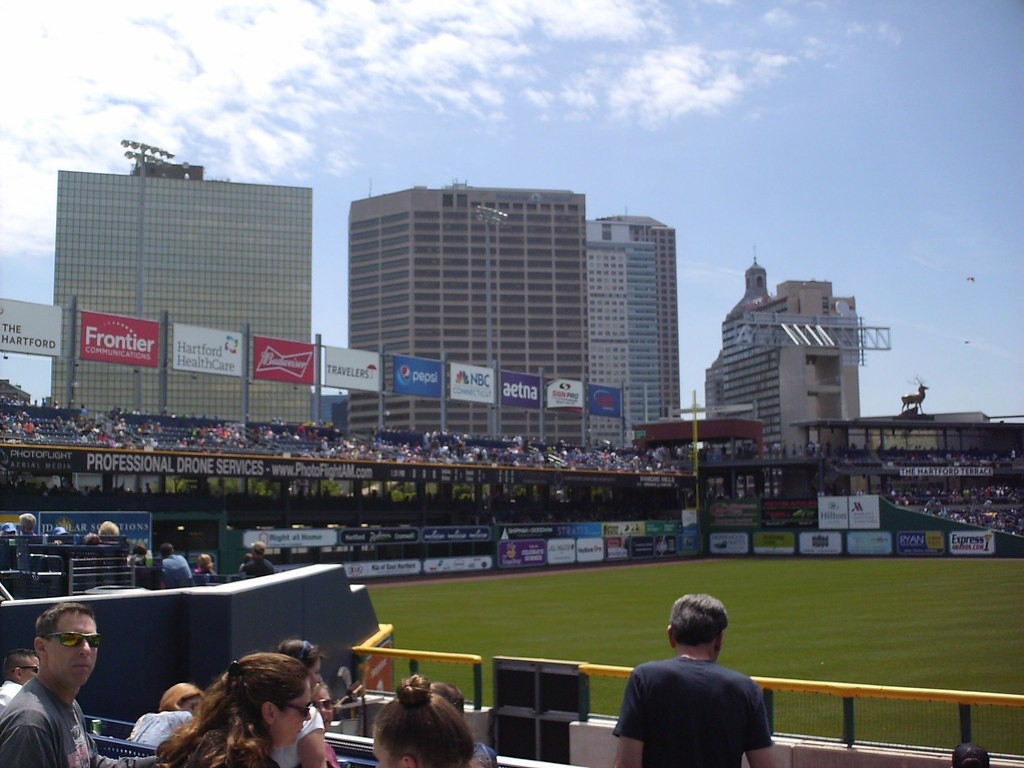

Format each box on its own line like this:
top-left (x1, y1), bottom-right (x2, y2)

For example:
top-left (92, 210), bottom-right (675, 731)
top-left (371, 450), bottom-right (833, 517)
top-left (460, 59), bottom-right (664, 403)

top-left (901, 376), bottom-right (930, 413)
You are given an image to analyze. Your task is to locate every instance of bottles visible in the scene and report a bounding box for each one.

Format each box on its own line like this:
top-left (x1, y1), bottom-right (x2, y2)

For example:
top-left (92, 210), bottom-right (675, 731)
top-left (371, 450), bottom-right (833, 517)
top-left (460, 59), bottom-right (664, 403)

top-left (91, 719), bottom-right (102, 736)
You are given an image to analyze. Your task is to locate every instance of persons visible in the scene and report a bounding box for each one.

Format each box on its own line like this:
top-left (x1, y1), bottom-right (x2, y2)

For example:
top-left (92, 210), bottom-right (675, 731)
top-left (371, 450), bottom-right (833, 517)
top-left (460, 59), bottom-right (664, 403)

top-left (128, 683), bottom-right (206, 746)
top-left (0, 395), bottom-right (1024, 594)
top-left (0, 602), bottom-right (159, 768)
top-left (952, 742), bottom-right (990, 768)
top-left (612, 593), bottom-right (780, 768)
top-left (373, 674), bottom-right (498, 768)
top-left (156, 652), bottom-right (311, 768)
top-left (266, 638), bottom-right (341, 768)
top-left (0, 648), bottom-right (40, 714)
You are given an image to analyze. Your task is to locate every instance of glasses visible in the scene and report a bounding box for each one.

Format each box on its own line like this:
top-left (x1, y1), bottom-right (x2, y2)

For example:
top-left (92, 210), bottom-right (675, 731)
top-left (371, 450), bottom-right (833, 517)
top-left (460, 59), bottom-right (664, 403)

top-left (12, 665), bottom-right (38, 673)
top-left (297, 640), bottom-right (311, 660)
top-left (266, 698), bottom-right (311, 717)
top-left (314, 699), bottom-right (334, 711)
top-left (43, 631), bottom-right (101, 648)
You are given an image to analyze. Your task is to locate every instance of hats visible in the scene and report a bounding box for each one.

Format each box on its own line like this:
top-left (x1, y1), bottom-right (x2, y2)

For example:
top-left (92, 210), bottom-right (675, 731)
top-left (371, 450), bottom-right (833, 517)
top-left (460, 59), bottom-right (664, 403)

top-left (251, 541), bottom-right (265, 552)
top-left (1, 523), bottom-right (16, 533)
top-left (52, 527), bottom-right (68, 534)
top-left (953, 743), bottom-right (989, 768)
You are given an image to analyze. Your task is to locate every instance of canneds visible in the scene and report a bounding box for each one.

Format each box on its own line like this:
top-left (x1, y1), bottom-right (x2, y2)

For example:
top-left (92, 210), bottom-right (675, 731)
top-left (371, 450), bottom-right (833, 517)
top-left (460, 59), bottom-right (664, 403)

top-left (91, 720), bottom-right (103, 736)
